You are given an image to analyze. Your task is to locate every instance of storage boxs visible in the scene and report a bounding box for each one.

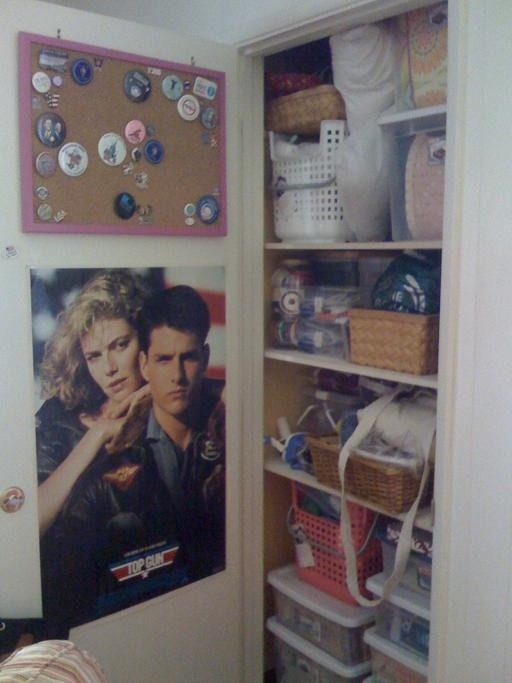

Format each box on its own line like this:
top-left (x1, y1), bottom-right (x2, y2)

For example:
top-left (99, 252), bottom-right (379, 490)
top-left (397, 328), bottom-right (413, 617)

top-left (265, 527), bottom-right (433, 683)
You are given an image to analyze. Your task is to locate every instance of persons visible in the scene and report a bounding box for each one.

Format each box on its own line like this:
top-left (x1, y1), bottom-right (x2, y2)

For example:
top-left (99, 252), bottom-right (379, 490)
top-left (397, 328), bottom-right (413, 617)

top-left (34, 268), bottom-right (227, 631)
top-left (42, 280), bottom-right (232, 594)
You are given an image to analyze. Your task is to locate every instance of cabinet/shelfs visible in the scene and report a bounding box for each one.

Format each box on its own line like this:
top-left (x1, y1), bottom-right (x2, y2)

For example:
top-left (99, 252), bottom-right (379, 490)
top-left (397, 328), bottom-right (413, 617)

top-left (0, 2), bottom-right (469, 682)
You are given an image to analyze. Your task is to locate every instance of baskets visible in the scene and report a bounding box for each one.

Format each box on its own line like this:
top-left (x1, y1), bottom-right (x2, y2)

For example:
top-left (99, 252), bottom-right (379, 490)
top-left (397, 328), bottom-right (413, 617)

top-left (291, 480), bottom-right (382, 606)
top-left (346, 309), bottom-right (439, 375)
top-left (265, 84), bottom-right (350, 243)
top-left (307, 436), bottom-right (428, 514)
